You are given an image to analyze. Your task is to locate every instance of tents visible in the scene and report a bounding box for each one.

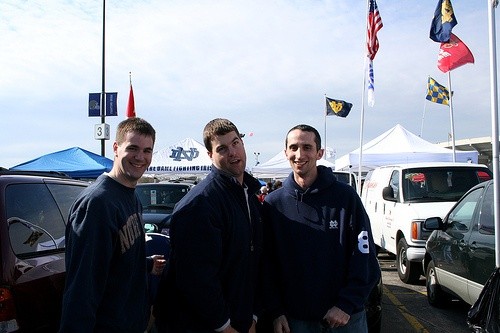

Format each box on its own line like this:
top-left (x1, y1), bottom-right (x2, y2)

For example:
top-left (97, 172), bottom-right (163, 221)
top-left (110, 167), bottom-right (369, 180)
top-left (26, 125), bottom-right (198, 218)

top-left (142, 136), bottom-right (248, 176)
top-left (9, 147), bottom-right (114, 181)
top-left (252, 147), bottom-right (335, 181)
top-left (334, 124), bottom-right (479, 202)
top-left (35, 236), bottom-right (65, 252)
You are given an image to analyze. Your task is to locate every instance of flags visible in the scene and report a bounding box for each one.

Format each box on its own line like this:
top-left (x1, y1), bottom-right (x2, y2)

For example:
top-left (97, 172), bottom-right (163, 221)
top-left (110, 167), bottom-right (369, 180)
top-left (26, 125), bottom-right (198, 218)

top-left (326, 97), bottom-right (354, 119)
top-left (428, 0), bottom-right (458, 44)
top-left (23, 231), bottom-right (45, 247)
top-left (437, 33), bottom-right (474, 73)
top-left (126, 83), bottom-right (136, 119)
top-left (366, 0), bottom-right (384, 109)
top-left (424, 77), bottom-right (455, 108)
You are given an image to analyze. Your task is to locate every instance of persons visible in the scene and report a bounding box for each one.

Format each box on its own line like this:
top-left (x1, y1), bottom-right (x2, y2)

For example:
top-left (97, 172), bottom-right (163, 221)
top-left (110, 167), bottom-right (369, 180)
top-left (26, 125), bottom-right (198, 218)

top-left (168, 115), bottom-right (271, 333)
top-left (65, 115), bottom-right (155, 333)
top-left (262, 125), bottom-right (383, 333)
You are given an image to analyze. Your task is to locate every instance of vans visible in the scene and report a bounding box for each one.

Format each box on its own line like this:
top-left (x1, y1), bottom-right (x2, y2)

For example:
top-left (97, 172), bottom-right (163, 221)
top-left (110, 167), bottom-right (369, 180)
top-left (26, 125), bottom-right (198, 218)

top-left (331, 170), bottom-right (358, 196)
top-left (361, 162), bottom-right (494, 284)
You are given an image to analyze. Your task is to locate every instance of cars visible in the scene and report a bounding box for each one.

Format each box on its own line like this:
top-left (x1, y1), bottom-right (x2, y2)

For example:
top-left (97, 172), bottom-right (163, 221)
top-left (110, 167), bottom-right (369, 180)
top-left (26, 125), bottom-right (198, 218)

top-left (422, 179), bottom-right (496, 311)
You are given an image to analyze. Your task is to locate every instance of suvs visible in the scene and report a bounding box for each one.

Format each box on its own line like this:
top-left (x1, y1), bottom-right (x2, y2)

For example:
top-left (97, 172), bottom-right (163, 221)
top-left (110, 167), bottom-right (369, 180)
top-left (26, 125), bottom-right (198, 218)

top-left (0, 172), bottom-right (92, 333)
top-left (134, 181), bottom-right (200, 236)
